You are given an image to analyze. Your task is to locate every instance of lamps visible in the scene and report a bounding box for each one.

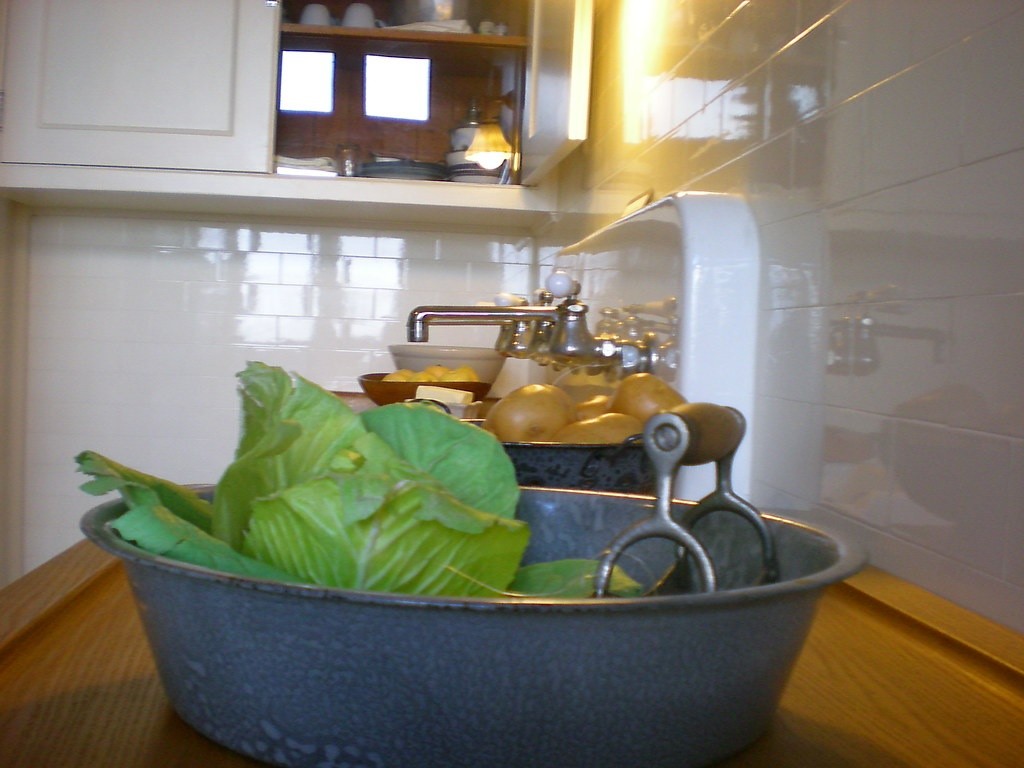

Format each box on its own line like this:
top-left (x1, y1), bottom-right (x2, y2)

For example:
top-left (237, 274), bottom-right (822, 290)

top-left (464, 88), bottom-right (519, 171)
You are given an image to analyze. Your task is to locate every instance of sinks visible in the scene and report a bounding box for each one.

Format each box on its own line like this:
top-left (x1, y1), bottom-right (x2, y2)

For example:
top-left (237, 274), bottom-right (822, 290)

top-left (388, 343), bottom-right (505, 383)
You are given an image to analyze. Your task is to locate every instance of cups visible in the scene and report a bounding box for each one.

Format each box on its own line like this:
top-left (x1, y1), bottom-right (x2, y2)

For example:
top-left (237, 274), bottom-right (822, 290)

top-left (341, 3), bottom-right (384, 28)
top-left (299, 3), bottom-right (335, 26)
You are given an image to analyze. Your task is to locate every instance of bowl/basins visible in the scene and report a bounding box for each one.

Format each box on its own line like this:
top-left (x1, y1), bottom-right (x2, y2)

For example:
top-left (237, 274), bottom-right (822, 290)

top-left (358, 374), bottom-right (490, 407)
top-left (445, 124), bottom-right (511, 186)
top-left (500, 438), bottom-right (684, 496)
top-left (80, 482), bottom-right (870, 768)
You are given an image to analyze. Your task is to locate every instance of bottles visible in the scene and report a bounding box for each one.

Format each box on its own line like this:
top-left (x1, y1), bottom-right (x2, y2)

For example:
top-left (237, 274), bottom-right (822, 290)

top-left (336, 143), bottom-right (360, 176)
top-left (478, 21), bottom-right (496, 35)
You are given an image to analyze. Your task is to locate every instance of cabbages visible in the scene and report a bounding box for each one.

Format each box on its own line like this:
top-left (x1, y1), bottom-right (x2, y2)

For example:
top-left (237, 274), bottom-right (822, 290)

top-left (74, 361), bottom-right (641, 600)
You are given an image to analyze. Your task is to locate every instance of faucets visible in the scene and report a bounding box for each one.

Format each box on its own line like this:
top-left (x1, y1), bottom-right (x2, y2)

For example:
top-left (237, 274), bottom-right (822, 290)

top-left (404, 297), bottom-right (602, 359)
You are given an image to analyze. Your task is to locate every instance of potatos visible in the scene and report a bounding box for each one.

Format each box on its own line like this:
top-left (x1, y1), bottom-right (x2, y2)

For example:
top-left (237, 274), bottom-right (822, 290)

top-left (382, 364), bottom-right (480, 383)
top-left (489, 372), bottom-right (686, 444)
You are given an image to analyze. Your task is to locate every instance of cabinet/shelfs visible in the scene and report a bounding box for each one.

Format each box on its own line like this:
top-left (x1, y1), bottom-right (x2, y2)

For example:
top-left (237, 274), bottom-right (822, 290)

top-left (1, 0), bottom-right (595, 235)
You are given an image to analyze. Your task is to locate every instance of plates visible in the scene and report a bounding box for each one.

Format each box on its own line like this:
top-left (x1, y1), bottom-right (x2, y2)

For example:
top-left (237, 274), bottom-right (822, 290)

top-left (356, 158), bottom-right (444, 180)
